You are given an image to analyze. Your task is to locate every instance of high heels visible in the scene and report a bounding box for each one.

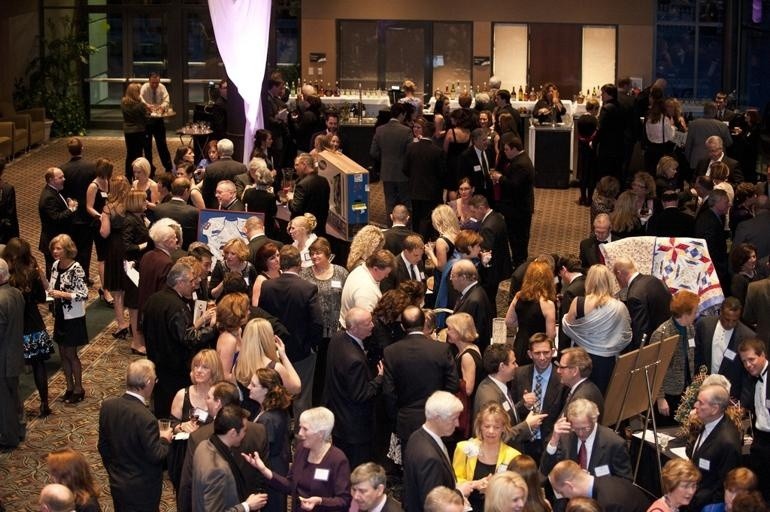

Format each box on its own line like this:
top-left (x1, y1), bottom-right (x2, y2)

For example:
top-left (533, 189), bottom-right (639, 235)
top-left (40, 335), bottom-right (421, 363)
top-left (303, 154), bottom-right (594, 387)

top-left (65, 390), bottom-right (86, 403)
top-left (99, 288), bottom-right (105, 300)
top-left (41, 402), bottom-right (53, 416)
top-left (61, 389), bottom-right (74, 401)
top-left (104, 296), bottom-right (116, 305)
top-left (111, 327), bottom-right (128, 339)
top-left (131, 346), bottom-right (148, 356)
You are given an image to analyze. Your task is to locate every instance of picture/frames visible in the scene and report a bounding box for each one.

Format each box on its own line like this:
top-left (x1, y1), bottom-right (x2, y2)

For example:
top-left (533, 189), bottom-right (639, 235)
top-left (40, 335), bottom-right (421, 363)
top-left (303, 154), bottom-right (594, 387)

top-left (198, 207), bottom-right (264, 274)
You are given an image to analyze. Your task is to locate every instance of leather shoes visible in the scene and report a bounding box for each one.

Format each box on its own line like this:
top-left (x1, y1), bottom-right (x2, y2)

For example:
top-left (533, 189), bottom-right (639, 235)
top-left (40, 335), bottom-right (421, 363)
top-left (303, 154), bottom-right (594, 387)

top-left (166, 167), bottom-right (172, 174)
top-left (584, 199), bottom-right (591, 208)
top-left (85, 278), bottom-right (95, 285)
top-left (1, 443), bottom-right (16, 449)
top-left (581, 198), bottom-right (587, 205)
top-left (151, 169), bottom-right (156, 180)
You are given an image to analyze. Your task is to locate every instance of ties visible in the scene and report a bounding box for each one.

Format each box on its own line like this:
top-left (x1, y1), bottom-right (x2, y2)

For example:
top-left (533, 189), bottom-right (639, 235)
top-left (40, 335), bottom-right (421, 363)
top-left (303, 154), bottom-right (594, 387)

top-left (530, 374), bottom-right (542, 440)
top-left (577, 440), bottom-right (587, 471)
top-left (695, 426), bottom-right (705, 454)
top-left (562, 392), bottom-right (572, 413)
top-left (153, 89), bottom-right (157, 104)
top-left (481, 152), bottom-right (489, 178)
top-left (444, 444), bottom-right (458, 483)
top-left (410, 265), bottom-right (418, 281)
top-left (718, 109), bottom-right (723, 120)
top-left (456, 292), bottom-right (464, 309)
top-left (508, 390), bottom-right (519, 420)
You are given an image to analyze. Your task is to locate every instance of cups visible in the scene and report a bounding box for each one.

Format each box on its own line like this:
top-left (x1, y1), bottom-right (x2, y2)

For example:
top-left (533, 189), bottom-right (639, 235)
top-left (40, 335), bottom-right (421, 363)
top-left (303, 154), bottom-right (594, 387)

top-left (159, 418), bottom-right (170, 432)
top-left (186, 122), bottom-right (211, 134)
top-left (189, 408), bottom-right (199, 423)
top-left (661, 432), bottom-right (669, 451)
top-left (483, 249), bottom-right (492, 268)
top-left (208, 300), bottom-right (215, 310)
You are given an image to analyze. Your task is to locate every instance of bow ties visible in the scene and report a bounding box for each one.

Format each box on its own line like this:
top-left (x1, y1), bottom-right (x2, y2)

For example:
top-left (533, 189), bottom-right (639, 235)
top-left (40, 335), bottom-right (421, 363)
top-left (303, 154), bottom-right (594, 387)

top-left (597, 240), bottom-right (609, 244)
top-left (755, 374), bottom-right (765, 384)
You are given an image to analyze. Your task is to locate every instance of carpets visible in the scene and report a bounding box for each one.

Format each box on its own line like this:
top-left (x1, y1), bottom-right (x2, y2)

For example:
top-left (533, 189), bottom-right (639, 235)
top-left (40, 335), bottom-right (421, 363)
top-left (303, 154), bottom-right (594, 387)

top-left (492, 187), bottom-right (592, 292)
top-left (0, 136), bottom-right (193, 354)
top-left (0, 310), bottom-right (301, 512)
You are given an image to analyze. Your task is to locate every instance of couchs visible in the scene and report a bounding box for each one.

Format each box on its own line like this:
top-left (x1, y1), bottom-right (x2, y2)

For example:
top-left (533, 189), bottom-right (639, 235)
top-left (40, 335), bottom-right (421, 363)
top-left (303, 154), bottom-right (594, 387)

top-left (0, 121), bottom-right (15, 163)
top-left (4, 101), bottom-right (45, 148)
top-left (1, 101), bottom-right (29, 158)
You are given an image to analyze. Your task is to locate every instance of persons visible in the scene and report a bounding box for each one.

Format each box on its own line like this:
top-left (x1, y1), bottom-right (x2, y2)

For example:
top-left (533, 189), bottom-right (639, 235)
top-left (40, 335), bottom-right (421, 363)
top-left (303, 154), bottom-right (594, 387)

top-left (398, 391), bottom-right (460, 512)
top-left (707, 162), bottom-right (735, 230)
top-left (613, 257), bottom-right (671, 350)
top-left (446, 180), bottom-right (476, 221)
top-left (428, 207), bottom-right (464, 271)
top-left (549, 402), bottom-right (634, 480)
top-left (431, 231), bottom-right (494, 329)
top-left (216, 272), bottom-right (249, 297)
top-left (175, 255), bottom-right (203, 317)
top-left (575, 102), bottom-right (599, 205)
top-left (556, 256), bottom-right (587, 317)
top-left (248, 370), bottom-right (294, 512)
top-left (81, 158), bottom-right (114, 307)
top-left (608, 193), bottom-right (644, 238)
top-left (498, 115), bottom-right (516, 141)
top-left (478, 111), bottom-right (497, 138)
top-left (740, 257), bottom-right (770, 341)
top-left (590, 177), bottom-right (620, 238)
top-left (712, 93), bottom-right (737, 123)
top-left (667, 386), bottom-right (741, 468)
top-left (728, 242), bottom-right (755, 300)
top-left (232, 319), bottom-right (301, 409)
top-left (38, 168), bottom-right (76, 296)
top-left (243, 168), bottom-right (279, 233)
top-left (474, 92), bottom-right (493, 128)
top-left (694, 190), bottom-right (731, 279)
top-left (590, 85), bottom-right (626, 187)
top-left (234, 159), bottom-right (267, 197)
top-left (0, 238), bottom-right (52, 415)
top-left (157, 178), bottom-right (199, 250)
top-left (381, 205), bottom-right (424, 255)
top-left (97, 359), bottom-right (173, 512)
top-left (630, 171), bottom-right (658, 205)
top-left (738, 109), bottom-right (759, 181)
top-left (459, 130), bottom-right (495, 207)
top-left (215, 181), bottom-right (244, 212)
top-left (121, 82), bottom-right (148, 180)
top-left (39, 484), bottom-right (76, 512)
top-left (510, 335), bottom-right (569, 443)
top-left (242, 407), bottom-right (350, 512)
top-left (323, 131), bottom-right (338, 151)
top-left (0, 260), bottom-right (27, 452)
top-left (400, 278), bottom-right (427, 309)
top-left (423, 486), bottom-right (471, 512)
top-left (451, 92), bottom-right (470, 108)
top-left (565, 495), bottom-right (603, 512)
top-left (447, 261), bottom-right (494, 362)
top-left (663, 97), bottom-right (686, 181)
top-left (249, 131), bottom-right (273, 172)
top-left (484, 76), bottom-right (503, 102)
top-left (127, 157), bottom-right (158, 215)
top-left (505, 263), bottom-right (557, 364)
top-left (736, 196), bottom-right (770, 256)
top-left (561, 264), bottom-right (632, 397)
top-left (101, 176), bottom-right (133, 338)
top-left (493, 133), bottom-right (535, 266)
top-left (260, 245), bottom-right (323, 407)
top-left (47, 448), bottom-right (103, 512)
top-left (120, 192), bottom-right (155, 356)
top-left (654, 156), bottom-right (679, 191)
top-left (637, 79), bottom-right (668, 139)
top-left (139, 262), bottom-right (216, 415)
top-left (243, 218), bottom-right (282, 266)
top-left (192, 406), bottom-right (267, 512)
top-left (447, 313), bottom-right (486, 401)
top-left (386, 306), bottom-right (460, 428)
top-left (211, 239), bottom-right (257, 293)
top-left (316, 114), bottom-right (338, 147)
top-left (297, 84), bottom-right (325, 146)
top-left (554, 351), bottom-right (607, 420)
top-left (289, 213), bottom-right (317, 268)
top-left (168, 147), bottom-right (194, 178)
top-left (288, 154), bottom-right (331, 236)
top-left (438, 109), bottom-right (474, 165)
top-left (179, 382), bottom-right (266, 512)
top-left (371, 103), bottom-right (415, 219)
top-left (643, 461), bottom-right (700, 512)
top-left (686, 101), bottom-right (733, 174)
top-left (701, 468), bottom-right (756, 512)
top-left (56, 139), bottom-right (94, 286)
top-left (139, 71), bottom-right (173, 171)
top-left (45, 234), bottom-right (85, 404)
top-left (473, 345), bottom-right (548, 445)
top-left (188, 241), bottom-right (213, 301)
top-left (379, 236), bottom-right (426, 293)
top-left (493, 90), bottom-right (524, 135)
top-left (432, 95), bottom-right (450, 140)
top-left (213, 294), bottom-right (249, 378)
top-left (649, 291), bottom-right (700, 419)
top-left (737, 338), bottom-right (770, 503)
top-left (172, 164), bottom-right (204, 209)
top-left (302, 239), bottom-right (349, 344)
top-left (338, 250), bottom-right (394, 328)
top-left (531, 84), bottom-right (566, 126)
top-left (250, 245), bottom-right (282, 308)
top-left (579, 212), bottom-right (621, 267)
top-left (1, 189), bottom-right (14, 244)
top-left (169, 350), bottom-right (223, 512)
top-left (349, 462), bottom-right (404, 512)
top-left (264, 77), bottom-right (288, 123)
top-left (695, 135), bottom-right (744, 185)
top-left (398, 81), bottom-right (422, 120)
top-left (465, 195), bottom-right (511, 274)
top-left (455, 404), bottom-right (520, 512)
top-left (645, 88), bottom-right (675, 166)
top-left (484, 472), bottom-right (527, 512)
top-left (364, 284), bottom-right (426, 360)
top-left (645, 190), bottom-right (695, 238)
top-left (730, 182), bottom-right (759, 242)
top-left (201, 138), bottom-right (246, 206)
top-left (196, 141), bottom-right (219, 181)
top-left (506, 458), bottom-right (551, 512)
top-left (324, 308), bottom-right (383, 463)
top-left (405, 121), bottom-right (449, 237)
top-left (213, 81), bottom-right (231, 119)
top-left (344, 225), bottom-right (386, 272)
top-left (615, 78), bottom-right (635, 172)
top-left (549, 462), bottom-right (652, 512)
top-left (139, 227), bottom-right (178, 297)
top-left (694, 298), bottom-right (764, 398)
top-left (0, 155), bottom-right (20, 238)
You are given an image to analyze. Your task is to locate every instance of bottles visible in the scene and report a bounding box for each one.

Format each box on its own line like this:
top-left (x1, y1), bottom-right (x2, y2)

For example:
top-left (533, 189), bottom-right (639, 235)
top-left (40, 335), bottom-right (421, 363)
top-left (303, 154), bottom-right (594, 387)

top-left (451, 84), bottom-right (455, 99)
top-left (334, 81), bottom-right (340, 97)
top-left (524, 93), bottom-right (529, 101)
top-left (297, 78), bottom-right (301, 97)
top-left (530, 88), bottom-right (536, 101)
top-left (463, 85), bottom-right (467, 94)
top-left (434, 88), bottom-right (442, 100)
top-left (511, 87), bottom-right (516, 100)
top-left (301, 79), bottom-right (325, 97)
top-left (362, 105), bottom-right (365, 118)
top-left (475, 85), bottom-right (480, 96)
top-left (469, 86), bottom-right (474, 100)
top-left (285, 82), bottom-right (289, 89)
top-left (444, 84), bottom-right (450, 97)
top-left (291, 81), bottom-right (295, 97)
top-left (592, 87), bottom-right (597, 98)
top-left (598, 85), bottom-right (601, 101)
top-left (519, 85), bottom-right (524, 101)
top-left (349, 105), bottom-right (359, 118)
top-left (538, 85), bottom-right (543, 99)
top-left (586, 90), bottom-right (591, 101)
top-left (577, 92), bottom-right (584, 104)
top-left (482, 82), bottom-right (488, 93)
top-left (456, 81), bottom-right (461, 99)
top-left (326, 82), bottom-right (333, 96)
